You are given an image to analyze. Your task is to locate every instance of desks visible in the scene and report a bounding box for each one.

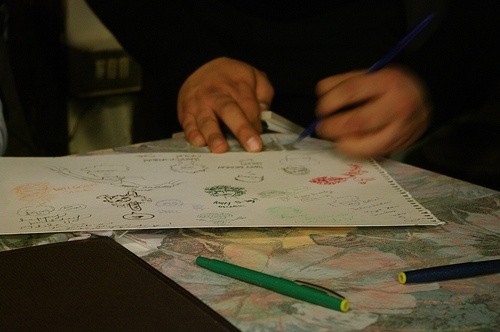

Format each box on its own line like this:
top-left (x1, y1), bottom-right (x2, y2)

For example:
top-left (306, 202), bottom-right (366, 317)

top-left (2, 130), bottom-right (500, 332)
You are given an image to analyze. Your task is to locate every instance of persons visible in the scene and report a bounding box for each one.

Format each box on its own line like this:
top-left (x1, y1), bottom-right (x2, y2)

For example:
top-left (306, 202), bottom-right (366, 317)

top-left (1, 0), bottom-right (73, 157)
top-left (83, 0), bottom-right (500, 197)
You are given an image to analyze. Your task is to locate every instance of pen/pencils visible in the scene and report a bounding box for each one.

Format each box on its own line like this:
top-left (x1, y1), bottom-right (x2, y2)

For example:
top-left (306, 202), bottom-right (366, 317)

top-left (196, 257), bottom-right (349, 312)
top-left (398, 260), bottom-right (500, 285)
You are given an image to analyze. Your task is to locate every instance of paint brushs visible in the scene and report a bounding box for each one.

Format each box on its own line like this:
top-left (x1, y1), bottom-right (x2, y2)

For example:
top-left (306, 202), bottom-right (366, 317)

top-left (282, 13), bottom-right (438, 151)
